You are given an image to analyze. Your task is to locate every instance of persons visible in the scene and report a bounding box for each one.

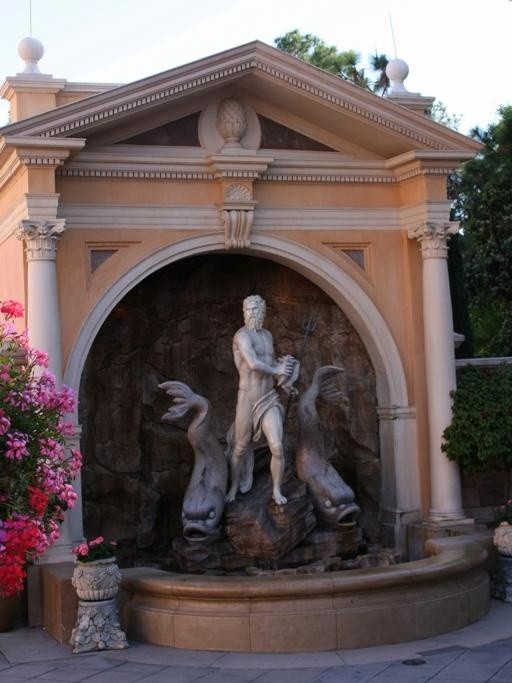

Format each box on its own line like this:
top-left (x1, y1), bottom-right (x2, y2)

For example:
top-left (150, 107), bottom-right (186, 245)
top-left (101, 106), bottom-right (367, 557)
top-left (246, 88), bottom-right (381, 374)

top-left (224, 295), bottom-right (299, 505)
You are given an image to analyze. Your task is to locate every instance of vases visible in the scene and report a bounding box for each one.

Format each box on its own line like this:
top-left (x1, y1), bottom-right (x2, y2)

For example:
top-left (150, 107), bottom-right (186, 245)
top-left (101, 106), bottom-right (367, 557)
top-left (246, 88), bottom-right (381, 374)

top-left (71, 556), bottom-right (123, 601)
top-left (1, 598), bottom-right (16, 633)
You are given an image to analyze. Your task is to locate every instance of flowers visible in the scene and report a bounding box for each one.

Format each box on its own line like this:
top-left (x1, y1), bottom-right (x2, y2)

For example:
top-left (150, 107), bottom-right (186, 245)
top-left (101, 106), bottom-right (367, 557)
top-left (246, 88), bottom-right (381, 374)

top-left (71, 535), bottom-right (119, 563)
top-left (1, 298), bottom-right (86, 598)
